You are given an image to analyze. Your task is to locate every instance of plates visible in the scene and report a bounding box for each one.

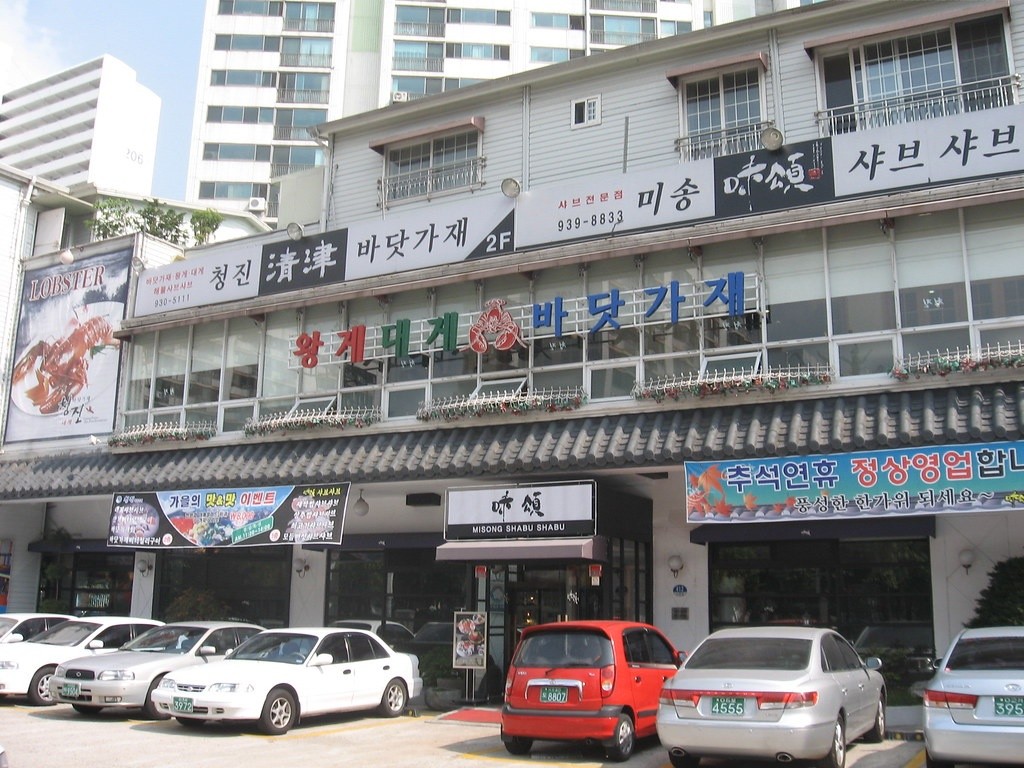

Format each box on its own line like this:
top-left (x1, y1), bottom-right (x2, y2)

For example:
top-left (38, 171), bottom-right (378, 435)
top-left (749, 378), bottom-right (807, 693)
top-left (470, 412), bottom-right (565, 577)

top-left (11, 300), bottom-right (124, 415)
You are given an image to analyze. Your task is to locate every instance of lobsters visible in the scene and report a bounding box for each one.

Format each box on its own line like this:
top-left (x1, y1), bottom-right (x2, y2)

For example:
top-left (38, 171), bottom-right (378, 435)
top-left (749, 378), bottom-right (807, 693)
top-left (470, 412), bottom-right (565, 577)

top-left (12, 316), bottom-right (120, 414)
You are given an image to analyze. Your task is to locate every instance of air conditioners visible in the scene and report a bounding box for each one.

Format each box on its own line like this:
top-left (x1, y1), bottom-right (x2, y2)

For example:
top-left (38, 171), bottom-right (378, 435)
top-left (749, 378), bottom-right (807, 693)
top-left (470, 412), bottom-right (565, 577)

top-left (393, 91), bottom-right (407, 103)
top-left (249, 197), bottom-right (265, 211)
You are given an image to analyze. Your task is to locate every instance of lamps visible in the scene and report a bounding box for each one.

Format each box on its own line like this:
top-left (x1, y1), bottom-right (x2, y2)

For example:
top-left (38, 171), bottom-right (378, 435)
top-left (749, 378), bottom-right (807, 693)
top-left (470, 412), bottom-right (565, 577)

top-left (668, 555), bottom-right (683, 578)
top-left (286, 222), bottom-right (305, 243)
top-left (60, 247), bottom-right (84, 265)
top-left (137, 560), bottom-right (152, 577)
top-left (131, 257), bottom-right (148, 272)
top-left (959, 548), bottom-right (974, 575)
top-left (293, 558), bottom-right (310, 579)
top-left (500, 177), bottom-right (520, 199)
top-left (760, 127), bottom-right (783, 150)
top-left (353, 489), bottom-right (369, 517)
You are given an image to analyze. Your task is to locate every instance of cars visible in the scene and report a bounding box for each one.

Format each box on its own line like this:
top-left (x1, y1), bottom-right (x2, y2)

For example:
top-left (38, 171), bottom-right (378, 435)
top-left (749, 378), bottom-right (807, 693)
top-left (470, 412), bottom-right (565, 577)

top-left (408, 621), bottom-right (455, 652)
top-left (328, 619), bottom-right (415, 649)
top-left (0, 612), bottom-right (164, 706)
top-left (151, 627), bottom-right (422, 734)
top-left (500, 620), bottom-right (687, 761)
top-left (656, 627), bottom-right (887, 768)
top-left (48, 621), bottom-right (266, 719)
top-left (922, 627), bottom-right (1024, 768)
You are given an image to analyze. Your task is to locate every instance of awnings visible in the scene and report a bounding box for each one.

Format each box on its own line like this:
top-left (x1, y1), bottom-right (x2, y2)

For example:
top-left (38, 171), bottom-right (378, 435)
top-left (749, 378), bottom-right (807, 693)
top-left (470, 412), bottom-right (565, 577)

top-left (436, 537), bottom-right (607, 561)
